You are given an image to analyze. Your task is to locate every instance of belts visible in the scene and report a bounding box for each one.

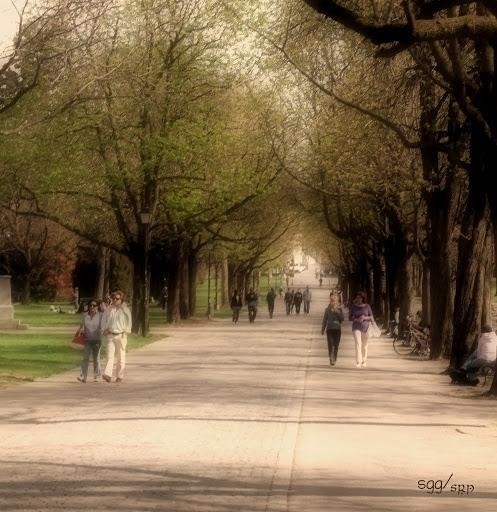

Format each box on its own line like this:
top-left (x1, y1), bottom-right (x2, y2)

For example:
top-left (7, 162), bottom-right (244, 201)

top-left (106, 329), bottom-right (125, 335)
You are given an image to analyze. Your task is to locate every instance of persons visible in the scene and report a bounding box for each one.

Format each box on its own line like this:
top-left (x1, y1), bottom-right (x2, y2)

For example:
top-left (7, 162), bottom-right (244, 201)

top-left (389, 305), bottom-right (401, 338)
top-left (349, 290), bottom-right (371, 365)
top-left (449, 324), bottom-right (497, 386)
top-left (100, 296), bottom-right (112, 311)
top-left (230, 289), bottom-right (242, 324)
top-left (403, 311), bottom-right (422, 348)
top-left (320, 289), bottom-right (343, 367)
top-left (266, 288), bottom-right (276, 318)
top-left (278, 285), bottom-right (311, 315)
top-left (76, 299), bottom-right (104, 382)
top-left (245, 287), bottom-right (256, 324)
top-left (101, 290), bottom-right (133, 383)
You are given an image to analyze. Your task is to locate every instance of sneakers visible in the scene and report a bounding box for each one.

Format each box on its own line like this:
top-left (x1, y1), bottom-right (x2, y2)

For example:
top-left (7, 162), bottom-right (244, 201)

top-left (93, 377), bottom-right (100, 382)
top-left (76, 373), bottom-right (88, 384)
top-left (102, 374), bottom-right (111, 383)
top-left (361, 361), bottom-right (368, 368)
top-left (115, 377), bottom-right (123, 383)
top-left (354, 362), bottom-right (362, 368)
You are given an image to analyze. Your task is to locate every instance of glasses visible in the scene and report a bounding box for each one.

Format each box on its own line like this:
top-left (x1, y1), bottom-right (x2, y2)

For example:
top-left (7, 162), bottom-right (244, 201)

top-left (112, 298), bottom-right (121, 300)
top-left (89, 304), bottom-right (98, 307)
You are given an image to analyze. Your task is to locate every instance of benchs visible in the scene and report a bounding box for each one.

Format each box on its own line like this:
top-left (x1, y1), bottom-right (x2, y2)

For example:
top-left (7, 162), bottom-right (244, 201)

top-left (479, 364), bottom-right (496, 385)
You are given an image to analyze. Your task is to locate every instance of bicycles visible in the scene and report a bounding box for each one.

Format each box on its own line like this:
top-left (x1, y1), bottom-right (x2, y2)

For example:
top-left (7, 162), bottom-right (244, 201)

top-left (393, 313), bottom-right (430, 354)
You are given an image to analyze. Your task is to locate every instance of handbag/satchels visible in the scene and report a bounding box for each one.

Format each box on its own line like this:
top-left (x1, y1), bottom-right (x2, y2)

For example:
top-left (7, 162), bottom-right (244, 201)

top-left (365, 317), bottom-right (381, 340)
top-left (69, 328), bottom-right (86, 351)
